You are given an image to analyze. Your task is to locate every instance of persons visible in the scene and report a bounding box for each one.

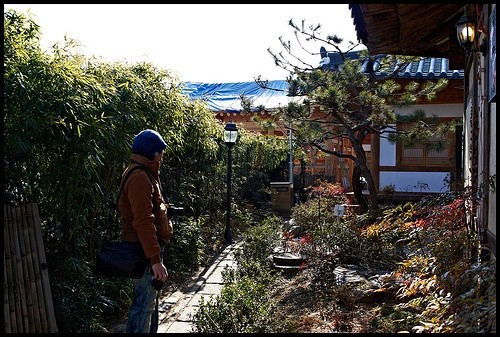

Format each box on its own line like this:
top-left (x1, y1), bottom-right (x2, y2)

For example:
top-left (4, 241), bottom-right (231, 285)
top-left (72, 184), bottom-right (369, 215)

top-left (119, 129), bottom-right (176, 334)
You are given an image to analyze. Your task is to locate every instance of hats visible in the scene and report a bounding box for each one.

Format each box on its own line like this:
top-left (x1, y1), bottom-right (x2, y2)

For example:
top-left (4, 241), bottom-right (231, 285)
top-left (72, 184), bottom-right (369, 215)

top-left (132, 129), bottom-right (168, 156)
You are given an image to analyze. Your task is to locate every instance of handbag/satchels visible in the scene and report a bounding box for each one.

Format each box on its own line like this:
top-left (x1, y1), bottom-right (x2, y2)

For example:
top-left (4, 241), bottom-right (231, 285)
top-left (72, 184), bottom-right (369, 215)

top-left (94, 239), bottom-right (149, 278)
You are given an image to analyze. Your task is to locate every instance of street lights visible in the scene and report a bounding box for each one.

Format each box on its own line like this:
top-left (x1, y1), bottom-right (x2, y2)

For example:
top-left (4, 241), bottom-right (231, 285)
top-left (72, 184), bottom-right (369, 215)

top-left (222, 123), bottom-right (238, 245)
